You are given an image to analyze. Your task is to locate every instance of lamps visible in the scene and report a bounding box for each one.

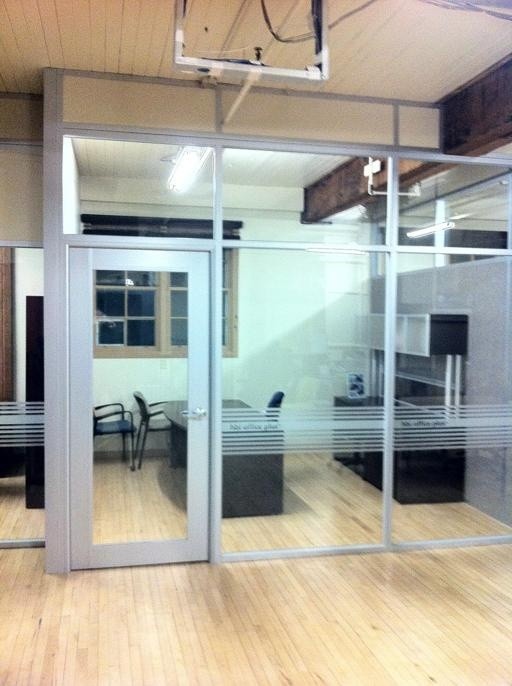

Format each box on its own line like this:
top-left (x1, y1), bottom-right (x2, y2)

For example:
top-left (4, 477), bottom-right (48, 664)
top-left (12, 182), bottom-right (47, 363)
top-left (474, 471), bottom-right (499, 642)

top-left (162, 145), bottom-right (212, 193)
top-left (407, 220), bottom-right (455, 241)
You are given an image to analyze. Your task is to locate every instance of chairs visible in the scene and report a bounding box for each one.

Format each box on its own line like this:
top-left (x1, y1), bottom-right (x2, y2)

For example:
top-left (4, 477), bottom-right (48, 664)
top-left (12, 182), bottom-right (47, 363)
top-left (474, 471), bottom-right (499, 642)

top-left (92, 402), bottom-right (137, 471)
top-left (132, 391), bottom-right (174, 469)
top-left (265, 391), bottom-right (287, 418)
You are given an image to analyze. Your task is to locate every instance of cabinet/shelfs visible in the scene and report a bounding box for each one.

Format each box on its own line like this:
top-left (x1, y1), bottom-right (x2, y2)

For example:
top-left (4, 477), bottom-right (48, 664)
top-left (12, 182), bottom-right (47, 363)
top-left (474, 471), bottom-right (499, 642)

top-left (332, 314), bottom-right (470, 505)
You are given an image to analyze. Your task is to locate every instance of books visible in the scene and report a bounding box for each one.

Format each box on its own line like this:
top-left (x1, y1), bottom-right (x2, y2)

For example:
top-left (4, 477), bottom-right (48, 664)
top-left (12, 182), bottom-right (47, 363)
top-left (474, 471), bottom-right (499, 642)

top-left (346, 372), bottom-right (368, 400)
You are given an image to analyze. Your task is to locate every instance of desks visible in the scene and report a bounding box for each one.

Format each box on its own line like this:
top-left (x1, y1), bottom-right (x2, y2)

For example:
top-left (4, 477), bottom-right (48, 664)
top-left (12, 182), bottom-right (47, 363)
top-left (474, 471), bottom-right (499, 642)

top-left (164, 398), bottom-right (286, 519)
